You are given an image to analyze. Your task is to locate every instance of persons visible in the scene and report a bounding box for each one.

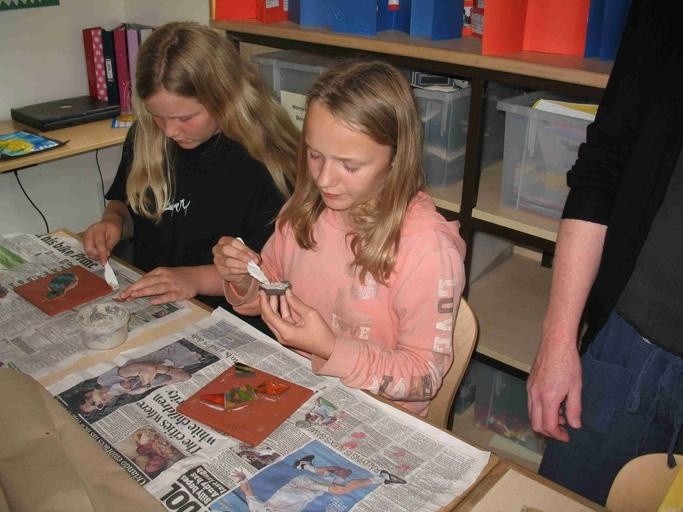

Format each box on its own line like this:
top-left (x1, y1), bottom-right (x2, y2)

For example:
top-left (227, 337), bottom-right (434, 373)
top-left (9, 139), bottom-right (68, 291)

top-left (81, 20), bottom-right (303, 344)
top-left (230, 454), bottom-right (409, 512)
top-left (133, 426), bottom-right (184, 480)
top-left (524, 0), bottom-right (683, 505)
top-left (65, 342), bottom-right (206, 415)
top-left (306, 396), bottom-right (338, 427)
top-left (216, 59), bottom-right (467, 418)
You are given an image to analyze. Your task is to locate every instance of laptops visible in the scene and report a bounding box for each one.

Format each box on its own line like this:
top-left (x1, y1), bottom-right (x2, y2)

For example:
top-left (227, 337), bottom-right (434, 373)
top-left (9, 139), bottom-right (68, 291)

top-left (11, 96), bottom-right (121, 132)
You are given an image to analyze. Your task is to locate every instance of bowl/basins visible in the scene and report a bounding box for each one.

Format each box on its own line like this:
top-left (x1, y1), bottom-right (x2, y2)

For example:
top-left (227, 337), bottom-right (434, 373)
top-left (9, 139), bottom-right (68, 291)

top-left (75, 301), bottom-right (130, 352)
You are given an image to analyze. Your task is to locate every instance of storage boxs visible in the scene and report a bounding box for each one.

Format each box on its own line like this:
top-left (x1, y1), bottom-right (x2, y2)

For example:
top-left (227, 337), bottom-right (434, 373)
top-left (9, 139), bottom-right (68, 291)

top-left (252, 49), bottom-right (333, 132)
top-left (453, 354), bottom-right (476, 416)
top-left (473, 357), bottom-right (554, 457)
top-left (497, 89), bottom-right (603, 219)
top-left (414, 73), bottom-right (514, 188)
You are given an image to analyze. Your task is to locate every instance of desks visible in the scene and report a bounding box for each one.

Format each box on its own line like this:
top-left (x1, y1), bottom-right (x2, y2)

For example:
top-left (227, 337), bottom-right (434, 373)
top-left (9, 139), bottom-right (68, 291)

top-left (0, 119), bottom-right (129, 174)
top-left (1, 226), bottom-right (498, 512)
top-left (456, 458), bottom-right (606, 512)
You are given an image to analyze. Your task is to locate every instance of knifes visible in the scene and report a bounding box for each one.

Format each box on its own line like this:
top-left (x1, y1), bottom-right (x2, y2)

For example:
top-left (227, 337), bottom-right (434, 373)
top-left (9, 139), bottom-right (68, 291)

top-left (103, 259), bottom-right (121, 290)
top-left (232, 236), bottom-right (271, 287)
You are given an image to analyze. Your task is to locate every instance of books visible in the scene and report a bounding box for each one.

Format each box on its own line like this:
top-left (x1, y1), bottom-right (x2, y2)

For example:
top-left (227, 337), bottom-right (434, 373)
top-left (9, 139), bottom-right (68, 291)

top-left (508, 97), bottom-right (603, 219)
top-left (86, 21), bottom-right (166, 114)
top-left (405, 72), bottom-right (503, 191)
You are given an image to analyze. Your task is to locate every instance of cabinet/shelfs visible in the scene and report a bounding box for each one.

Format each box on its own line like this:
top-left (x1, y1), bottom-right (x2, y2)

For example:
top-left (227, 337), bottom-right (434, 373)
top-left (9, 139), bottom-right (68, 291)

top-left (209, 15), bottom-right (616, 471)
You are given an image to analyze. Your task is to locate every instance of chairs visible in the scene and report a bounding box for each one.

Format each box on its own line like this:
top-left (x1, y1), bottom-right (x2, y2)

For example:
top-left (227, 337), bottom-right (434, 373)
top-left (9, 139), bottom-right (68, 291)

top-left (423, 296), bottom-right (479, 430)
top-left (607, 453), bottom-right (682, 511)
